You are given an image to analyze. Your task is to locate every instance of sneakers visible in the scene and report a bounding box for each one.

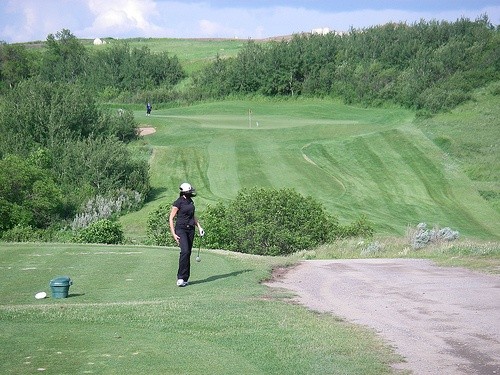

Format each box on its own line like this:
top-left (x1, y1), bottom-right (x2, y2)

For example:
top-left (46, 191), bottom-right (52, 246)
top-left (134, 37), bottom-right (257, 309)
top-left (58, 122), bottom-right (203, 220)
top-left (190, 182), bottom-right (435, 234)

top-left (177, 279), bottom-right (188, 286)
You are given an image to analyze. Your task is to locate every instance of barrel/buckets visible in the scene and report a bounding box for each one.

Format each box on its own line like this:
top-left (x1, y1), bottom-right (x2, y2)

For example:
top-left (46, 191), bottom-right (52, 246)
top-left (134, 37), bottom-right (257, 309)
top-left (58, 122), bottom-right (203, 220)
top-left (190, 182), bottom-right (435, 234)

top-left (51, 276), bottom-right (72, 299)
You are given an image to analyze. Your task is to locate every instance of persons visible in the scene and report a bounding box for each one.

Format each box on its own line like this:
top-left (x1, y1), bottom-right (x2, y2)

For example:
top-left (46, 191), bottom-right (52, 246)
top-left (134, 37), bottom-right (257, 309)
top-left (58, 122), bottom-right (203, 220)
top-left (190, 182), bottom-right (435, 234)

top-left (145, 101), bottom-right (153, 117)
top-left (170, 182), bottom-right (205, 286)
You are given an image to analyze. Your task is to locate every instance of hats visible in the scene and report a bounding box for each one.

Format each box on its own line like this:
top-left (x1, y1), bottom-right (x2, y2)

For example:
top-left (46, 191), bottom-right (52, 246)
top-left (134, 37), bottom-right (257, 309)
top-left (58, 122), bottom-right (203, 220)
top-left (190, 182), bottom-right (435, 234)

top-left (179, 183), bottom-right (195, 192)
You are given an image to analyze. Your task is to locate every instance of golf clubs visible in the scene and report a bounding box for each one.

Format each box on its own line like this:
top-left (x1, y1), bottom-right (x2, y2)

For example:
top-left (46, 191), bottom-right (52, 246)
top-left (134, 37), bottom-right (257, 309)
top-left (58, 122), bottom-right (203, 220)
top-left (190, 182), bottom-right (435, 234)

top-left (196, 231), bottom-right (203, 262)
